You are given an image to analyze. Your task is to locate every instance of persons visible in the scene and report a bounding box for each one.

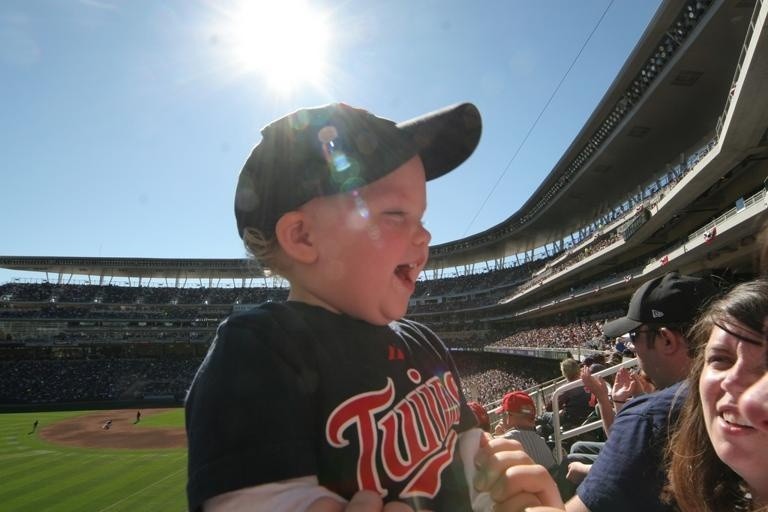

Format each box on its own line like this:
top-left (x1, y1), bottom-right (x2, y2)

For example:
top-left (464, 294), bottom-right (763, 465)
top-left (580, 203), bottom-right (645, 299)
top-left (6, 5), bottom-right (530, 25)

top-left (1, 282), bottom-right (290, 403)
top-left (738, 371), bottom-right (768, 433)
top-left (102, 420), bottom-right (112, 430)
top-left (137, 411), bottom-right (140, 421)
top-left (33, 420), bottom-right (38, 430)
top-left (185, 103), bottom-right (558, 512)
top-left (405, 249), bottom-right (656, 490)
top-left (565, 272), bottom-right (716, 512)
top-left (473, 280), bottom-right (768, 512)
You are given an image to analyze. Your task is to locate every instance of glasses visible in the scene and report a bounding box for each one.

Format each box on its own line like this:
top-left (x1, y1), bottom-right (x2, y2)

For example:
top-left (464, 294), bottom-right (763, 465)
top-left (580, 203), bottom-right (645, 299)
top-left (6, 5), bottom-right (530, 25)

top-left (627, 328), bottom-right (677, 340)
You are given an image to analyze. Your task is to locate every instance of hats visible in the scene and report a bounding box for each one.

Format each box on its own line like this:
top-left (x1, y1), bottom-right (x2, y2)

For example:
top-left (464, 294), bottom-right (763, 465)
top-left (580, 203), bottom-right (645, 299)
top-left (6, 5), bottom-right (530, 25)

top-left (494, 392), bottom-right (536, 416)
top-left (235, 102), bottom-right (481, 238)
top-left (601, 271), bottom-right (719, 338)
